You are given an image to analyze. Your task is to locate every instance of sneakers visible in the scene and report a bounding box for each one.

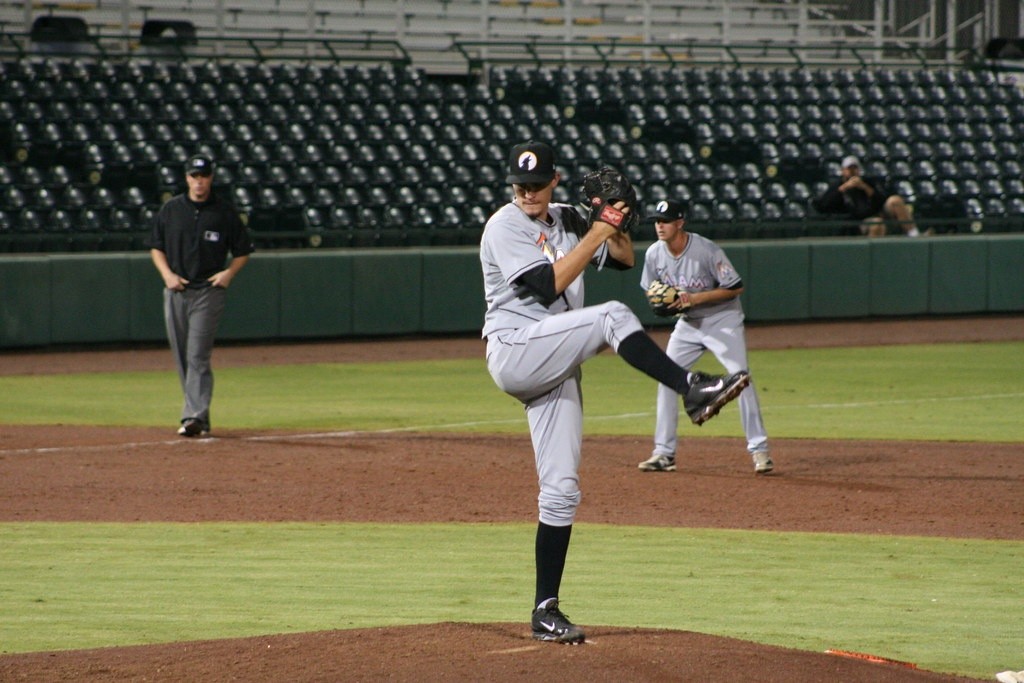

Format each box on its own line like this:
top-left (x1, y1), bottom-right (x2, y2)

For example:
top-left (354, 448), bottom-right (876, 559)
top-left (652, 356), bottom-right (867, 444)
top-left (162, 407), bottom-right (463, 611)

top-left (684, 371), bottom-right (749, 426)
top-left (531, 598), bottom-right (585, 644)
top-left (751, 451), bottom-right (773, 472)
top-left (639, 454), bottom-right (676, 472)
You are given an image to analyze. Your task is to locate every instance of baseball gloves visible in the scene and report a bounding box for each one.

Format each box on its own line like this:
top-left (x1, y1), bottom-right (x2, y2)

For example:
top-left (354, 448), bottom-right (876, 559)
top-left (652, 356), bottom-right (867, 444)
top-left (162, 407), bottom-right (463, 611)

top-left (646, 279), bottom-right (692, 319)
top-left (580, 162), bottom-right (640, 235)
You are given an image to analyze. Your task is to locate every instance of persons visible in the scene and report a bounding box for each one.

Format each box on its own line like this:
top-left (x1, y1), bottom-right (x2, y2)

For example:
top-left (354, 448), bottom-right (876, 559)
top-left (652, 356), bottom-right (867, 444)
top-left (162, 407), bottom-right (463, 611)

top-left (478, 143), bottom-right (750, 646)
top-left (635, 193), bottom-right (773, 475)
top-left (820, 155), bottom-right (919, 236)
top-left (147, 156), bottom-right (253, 439)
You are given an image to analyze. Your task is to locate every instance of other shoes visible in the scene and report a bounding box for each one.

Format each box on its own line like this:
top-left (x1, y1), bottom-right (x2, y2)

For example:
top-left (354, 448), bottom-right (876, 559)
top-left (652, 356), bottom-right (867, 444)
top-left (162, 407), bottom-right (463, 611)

top-left (177, 417), bottom-right (209, 436)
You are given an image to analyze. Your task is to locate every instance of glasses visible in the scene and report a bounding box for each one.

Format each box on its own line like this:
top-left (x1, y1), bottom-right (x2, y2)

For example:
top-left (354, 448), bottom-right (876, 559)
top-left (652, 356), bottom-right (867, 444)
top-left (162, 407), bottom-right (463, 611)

top-left (189, 173), bottom-right (210, 178)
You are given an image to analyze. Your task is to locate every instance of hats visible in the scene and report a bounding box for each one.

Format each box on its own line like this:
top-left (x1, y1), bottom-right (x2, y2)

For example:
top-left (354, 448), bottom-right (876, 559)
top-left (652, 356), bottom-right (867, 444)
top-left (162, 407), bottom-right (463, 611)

top-left (506, 141), bottom-right (555, 184)
top-left (647, 199), bottom-right (685, 221)
top-left (186, 155), bottom-right (213, 174)
top-left (842, 155), bottom-right (861, 167)
